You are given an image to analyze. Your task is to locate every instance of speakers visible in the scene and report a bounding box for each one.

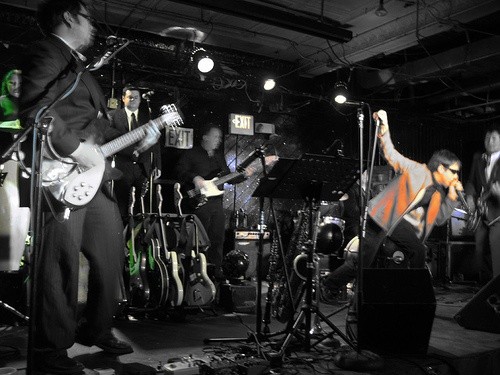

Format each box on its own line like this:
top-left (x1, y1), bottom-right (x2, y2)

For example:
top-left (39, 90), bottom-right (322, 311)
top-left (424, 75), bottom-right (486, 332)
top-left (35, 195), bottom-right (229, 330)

top-left (345, 267), bottom-right (438, 359)
top-left (453, 272), bottom-right (500, 333)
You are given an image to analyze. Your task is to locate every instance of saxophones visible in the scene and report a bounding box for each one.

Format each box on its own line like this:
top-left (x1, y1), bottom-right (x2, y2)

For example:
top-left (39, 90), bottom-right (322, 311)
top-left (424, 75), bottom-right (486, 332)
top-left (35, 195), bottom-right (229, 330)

top-left (271, 197), bottom-right (318, 323)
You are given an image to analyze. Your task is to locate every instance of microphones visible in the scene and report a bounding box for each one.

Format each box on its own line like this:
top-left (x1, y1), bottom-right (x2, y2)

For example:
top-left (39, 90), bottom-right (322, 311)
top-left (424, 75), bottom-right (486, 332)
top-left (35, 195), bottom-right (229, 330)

top-left (455, 184), bottom-right (471, 211)
top-left (105, 35), bottom-right (127, 50)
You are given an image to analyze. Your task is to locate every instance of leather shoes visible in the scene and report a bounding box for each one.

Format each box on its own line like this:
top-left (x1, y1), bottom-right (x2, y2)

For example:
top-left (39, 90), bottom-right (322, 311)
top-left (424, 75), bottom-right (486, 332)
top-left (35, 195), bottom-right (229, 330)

top-left (68, 357), bottom-right (100, 375)
top-left (74, 329), bottom-right (135, 354)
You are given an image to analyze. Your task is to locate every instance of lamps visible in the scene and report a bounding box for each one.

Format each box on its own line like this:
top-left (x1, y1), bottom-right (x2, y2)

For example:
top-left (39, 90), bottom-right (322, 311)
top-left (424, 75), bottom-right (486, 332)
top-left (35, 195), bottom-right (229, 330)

top-left (375, 0), bottom-right (388, 17)
top-left (257, 68), bottom-right (276, 91)
top-left (189, 43), bottom-right (215, 73)
top-left (334, 67), bottom-right (349, 104)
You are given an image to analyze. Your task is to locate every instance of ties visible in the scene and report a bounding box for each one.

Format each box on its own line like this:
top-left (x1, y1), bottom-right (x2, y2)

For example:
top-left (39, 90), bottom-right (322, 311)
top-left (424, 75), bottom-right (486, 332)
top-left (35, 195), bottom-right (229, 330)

top-left (131, 113), bottom-right (139, 146)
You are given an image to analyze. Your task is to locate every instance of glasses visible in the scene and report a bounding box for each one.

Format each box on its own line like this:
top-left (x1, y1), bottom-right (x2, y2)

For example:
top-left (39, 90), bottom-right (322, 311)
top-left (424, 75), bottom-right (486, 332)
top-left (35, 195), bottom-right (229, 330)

top-left (69, 8), bottom-right (97, 25)
top-left (444, 165), bottom-right (460, 175)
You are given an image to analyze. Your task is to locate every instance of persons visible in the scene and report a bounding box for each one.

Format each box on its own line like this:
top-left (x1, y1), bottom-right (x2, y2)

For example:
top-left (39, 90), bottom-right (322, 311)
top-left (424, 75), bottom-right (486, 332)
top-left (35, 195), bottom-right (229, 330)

top-left (318, 109), bottom-right (464, 305)
top-left (18, 0), bottom-right (162, 375)
top-left (106, 86), bottom-right (157, 220)
top-left (339, 169), bottom-right (373, 254)
top-left (181, 124), bottom-right (256, 283)
top-left (0, 69), bottom-right (26, 133)
top-left (464, 129), bottom-right (500, 278)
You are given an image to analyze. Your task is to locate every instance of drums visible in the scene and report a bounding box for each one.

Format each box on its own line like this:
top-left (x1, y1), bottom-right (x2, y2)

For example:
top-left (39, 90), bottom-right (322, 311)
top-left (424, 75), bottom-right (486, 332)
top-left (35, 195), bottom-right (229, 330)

top-left (343, 234), bottom-right (360, 261)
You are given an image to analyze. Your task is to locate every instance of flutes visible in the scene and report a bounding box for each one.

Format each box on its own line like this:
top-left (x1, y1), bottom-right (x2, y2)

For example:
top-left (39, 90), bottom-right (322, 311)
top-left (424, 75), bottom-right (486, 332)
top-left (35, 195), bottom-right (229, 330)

top-left (262, 229), bottom-right (281, 324)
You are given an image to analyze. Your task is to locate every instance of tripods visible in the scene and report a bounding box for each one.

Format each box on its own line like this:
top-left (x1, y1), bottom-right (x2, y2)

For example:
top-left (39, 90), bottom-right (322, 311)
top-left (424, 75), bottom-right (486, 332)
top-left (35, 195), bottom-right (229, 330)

top-left (202, 157), bottom-right (351, 355)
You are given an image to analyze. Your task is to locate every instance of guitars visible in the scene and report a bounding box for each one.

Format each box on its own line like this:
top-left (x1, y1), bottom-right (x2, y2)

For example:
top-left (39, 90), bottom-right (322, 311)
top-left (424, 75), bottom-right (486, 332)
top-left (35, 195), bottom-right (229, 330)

top-left (40, 103), bottom-right (184, 209)
top-left (127, 186), bottom-right (144, 293)
top-left (187, 155), bottom-right (281, 203)
top-left (467, 179), bottom-right (493, 233)
top-left (123, 178), bottom-right (216, 311)
